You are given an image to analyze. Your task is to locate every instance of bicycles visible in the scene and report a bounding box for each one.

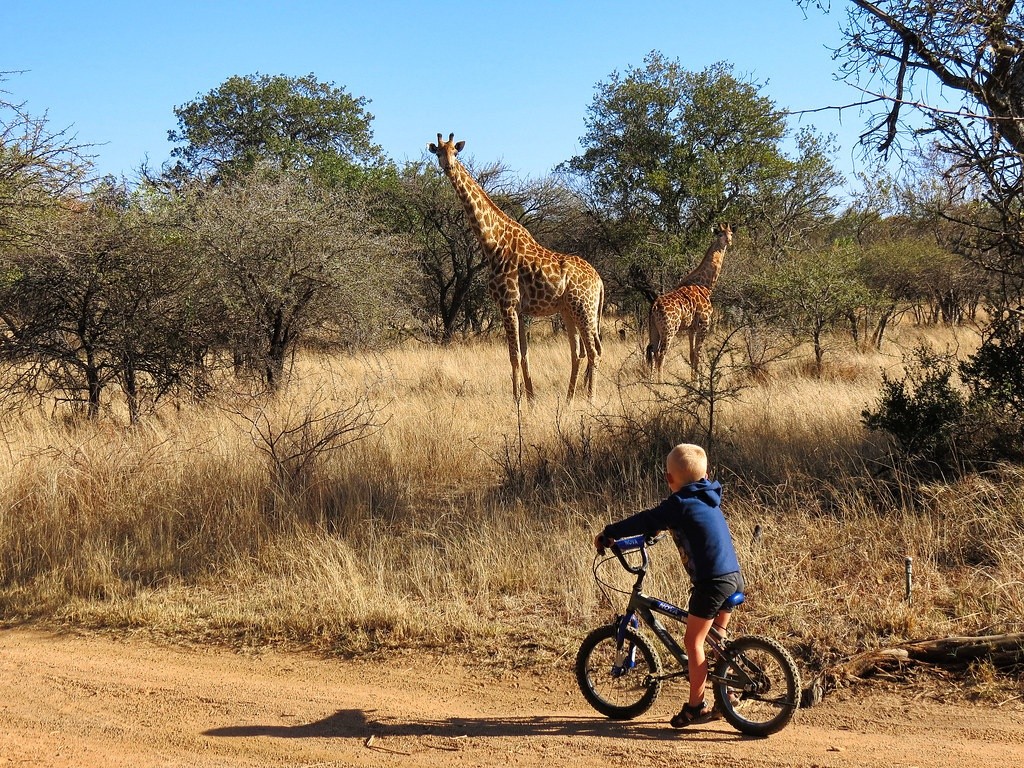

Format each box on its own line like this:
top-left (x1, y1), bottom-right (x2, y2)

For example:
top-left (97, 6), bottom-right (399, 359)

top-left (577, 523), bottom-right (800, 738)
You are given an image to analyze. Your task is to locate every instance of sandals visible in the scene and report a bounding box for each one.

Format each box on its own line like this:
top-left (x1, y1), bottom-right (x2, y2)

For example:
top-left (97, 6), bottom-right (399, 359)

top-left (670, 701), bottom-right (713, 728)
top-left (712, 690), bottom-right (740, 720)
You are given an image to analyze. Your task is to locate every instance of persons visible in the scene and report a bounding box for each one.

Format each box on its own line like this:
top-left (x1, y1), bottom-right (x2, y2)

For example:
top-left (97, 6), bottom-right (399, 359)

top-left (594, 442), bottom-right (744, 727)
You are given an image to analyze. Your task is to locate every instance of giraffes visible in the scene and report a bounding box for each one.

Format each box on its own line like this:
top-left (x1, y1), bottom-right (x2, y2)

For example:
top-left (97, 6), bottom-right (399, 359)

top-left (645, 222), bottom-right (733, 389)
top-left (427, 132), bottom-right (606, 411)
